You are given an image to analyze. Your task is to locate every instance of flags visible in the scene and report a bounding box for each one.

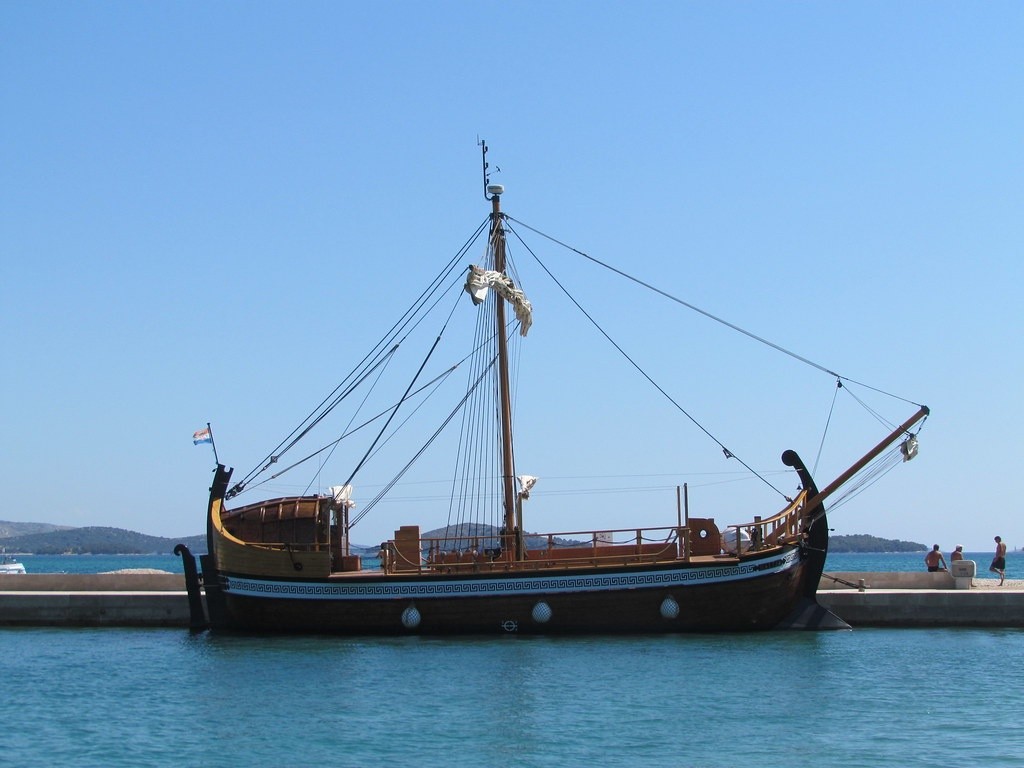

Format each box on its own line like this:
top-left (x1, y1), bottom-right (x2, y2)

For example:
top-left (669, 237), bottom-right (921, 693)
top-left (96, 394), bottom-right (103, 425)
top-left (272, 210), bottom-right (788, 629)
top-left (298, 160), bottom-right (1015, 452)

top-left (193, 428), bottom-right (213, 446)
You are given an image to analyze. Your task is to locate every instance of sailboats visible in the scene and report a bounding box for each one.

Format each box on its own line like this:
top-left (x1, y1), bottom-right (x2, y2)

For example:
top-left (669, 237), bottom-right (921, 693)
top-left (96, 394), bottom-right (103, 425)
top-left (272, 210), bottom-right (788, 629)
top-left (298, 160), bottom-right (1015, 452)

top-left (171, 139), bottom-right (931, 635)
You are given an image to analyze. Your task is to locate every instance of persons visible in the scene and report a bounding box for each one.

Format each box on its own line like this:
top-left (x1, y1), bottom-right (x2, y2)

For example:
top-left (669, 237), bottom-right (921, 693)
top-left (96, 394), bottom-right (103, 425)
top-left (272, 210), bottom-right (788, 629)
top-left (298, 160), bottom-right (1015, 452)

top-left (925, 544), bottom-right (948, 572)
top-left (989, 536), bottom-right (1006, 586)
top-left (950, 545), bottom-right (964, 563)
